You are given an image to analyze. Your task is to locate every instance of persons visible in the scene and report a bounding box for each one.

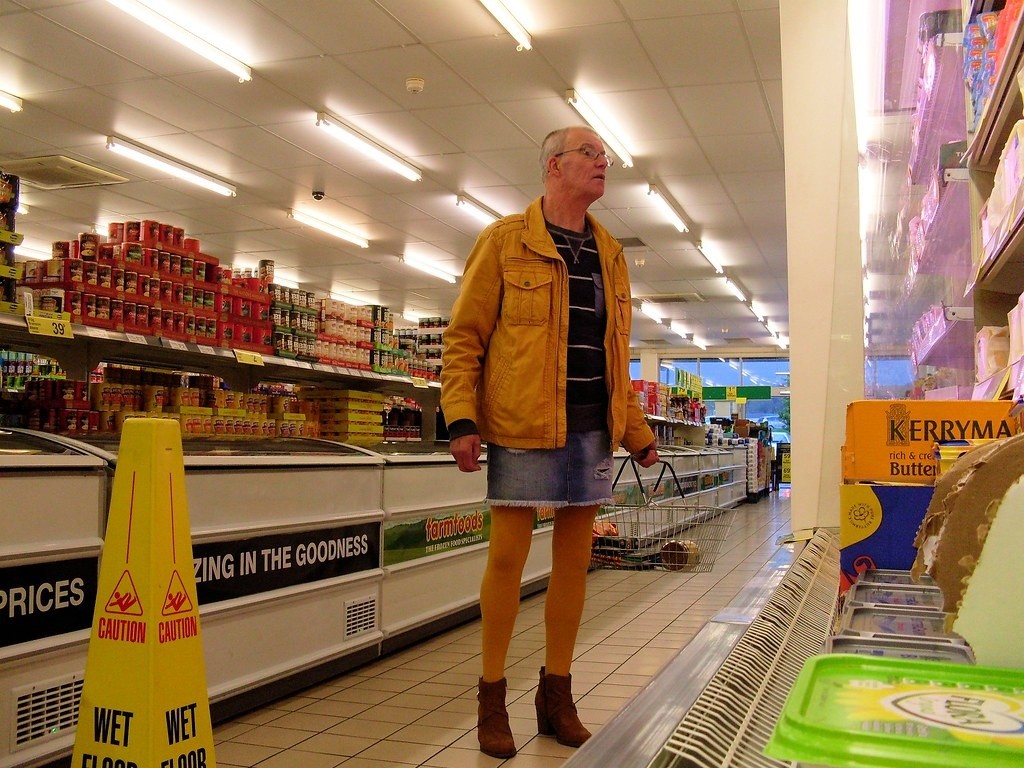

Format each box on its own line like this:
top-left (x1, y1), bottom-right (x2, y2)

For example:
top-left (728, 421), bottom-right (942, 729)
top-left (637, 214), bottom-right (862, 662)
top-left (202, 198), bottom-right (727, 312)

top-left (441, 126), bottom-right (659, 757)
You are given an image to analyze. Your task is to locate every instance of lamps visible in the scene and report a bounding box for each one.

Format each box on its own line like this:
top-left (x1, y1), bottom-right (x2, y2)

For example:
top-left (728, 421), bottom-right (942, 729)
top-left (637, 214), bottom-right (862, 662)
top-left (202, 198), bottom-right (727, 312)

top-left (748, 304), bottom-right (764, 322)
top-left (479, 0), bottom-right (532, 51)
top-left (666, 322), bottom-right (687, 338)
top-left (315, 113), bottom-right (422, 181)
top-left (287, 208), bottom-right (369, 248)
top-left (398, 254), bottom-right (456, 283)
top-left (565, 89), bottom-right (634, 168)
top-left (457, 195), bottom-right (499, 226)
top-left (648, 184), bottom-right (690, 232)
top-left (690, 338), bottom-right (707, 350)
top-left (727, 278), bottom-right (747, 301)
top-left (106, 136), bottom-right (236, 196)
top-left (764, 321), bottom-right (787, 336)
top-left (696, 240), bottom-right (724, 274)
top-left (107, 0), bottom-right (251, 81)
top-left (637, 301), bottom-right (662, 324)
top-left (776, 332), bottom-right (787, 350)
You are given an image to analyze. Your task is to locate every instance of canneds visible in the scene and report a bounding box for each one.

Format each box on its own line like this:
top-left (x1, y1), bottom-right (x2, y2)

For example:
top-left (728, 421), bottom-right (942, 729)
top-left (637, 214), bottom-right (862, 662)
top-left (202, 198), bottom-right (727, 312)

top-left (16, 220), bottom-right (451, 384)
top-left (21, 377), bottom-right (321, 440)
top-left (382, 410), bottom-right (421, 438)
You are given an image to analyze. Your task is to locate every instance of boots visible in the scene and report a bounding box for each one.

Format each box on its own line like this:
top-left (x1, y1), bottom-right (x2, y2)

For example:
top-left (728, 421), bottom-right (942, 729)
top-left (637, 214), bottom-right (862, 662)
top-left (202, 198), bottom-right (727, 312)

top-left (533, 665), bottom-right (593, 749)
top-left (477, 675), bottom-right (515, 759)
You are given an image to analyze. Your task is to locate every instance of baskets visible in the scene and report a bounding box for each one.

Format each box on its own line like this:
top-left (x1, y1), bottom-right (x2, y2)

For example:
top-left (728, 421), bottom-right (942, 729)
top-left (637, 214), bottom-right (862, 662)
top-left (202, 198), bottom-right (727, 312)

top-left (589, 457), bottom-right (736, 574)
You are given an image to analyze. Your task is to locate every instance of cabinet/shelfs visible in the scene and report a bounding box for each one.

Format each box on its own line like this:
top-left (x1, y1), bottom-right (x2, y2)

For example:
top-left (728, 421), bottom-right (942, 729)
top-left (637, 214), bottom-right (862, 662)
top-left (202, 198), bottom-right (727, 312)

top-left (964, 0), bottom-right (1024, 400)
top-left (0, 312), bottom-right (443, 441)
top-left (635, 414), bottom-right (706, 446)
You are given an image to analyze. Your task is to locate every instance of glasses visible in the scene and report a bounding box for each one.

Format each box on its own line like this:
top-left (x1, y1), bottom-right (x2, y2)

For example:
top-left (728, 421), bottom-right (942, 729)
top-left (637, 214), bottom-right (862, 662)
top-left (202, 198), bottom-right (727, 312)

top-left (555, 144), bottom-right (614, 168)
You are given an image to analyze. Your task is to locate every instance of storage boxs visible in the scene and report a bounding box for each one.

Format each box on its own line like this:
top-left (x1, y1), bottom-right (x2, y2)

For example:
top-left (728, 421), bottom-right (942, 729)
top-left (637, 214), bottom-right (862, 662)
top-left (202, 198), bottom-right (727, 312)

top-left (321, 413), bottom-right (382, 422)
top-left (320, 402), bottom-right (384, 412)
top-left (838, 400), bottom-right (1024, 614)
top-left (762, 651), bottom-right (1022, 766)
top-left (321, 424), bottom-right (383, 433)
top-left (631, 380), bottom-right (702, 417)
top-left (299, 389), bottom-right (384, 401)
top-left (212, 408), bottom-right (246, 417)
top-left (165, 406), bottom-right (212, 415)
top-left (322, 436), bottom-right (384, 442)
top-left (270, 413), bottom-right (306, 420)
top-left (706, 420), bottom-right (791, 491)
